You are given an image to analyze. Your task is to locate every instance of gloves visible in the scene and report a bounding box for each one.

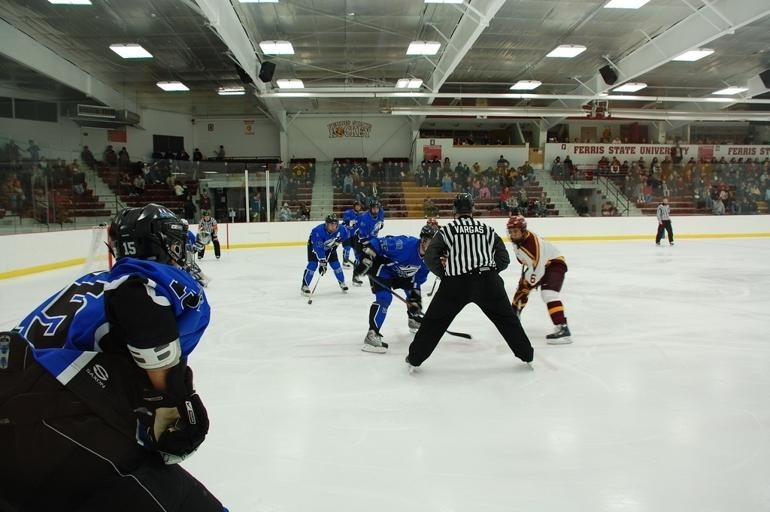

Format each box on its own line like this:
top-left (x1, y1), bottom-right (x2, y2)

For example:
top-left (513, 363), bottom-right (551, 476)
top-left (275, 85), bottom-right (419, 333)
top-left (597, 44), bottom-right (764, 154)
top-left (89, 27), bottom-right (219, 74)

top-left (135, 388), bottom-right (208, 468)
top-left (405, 295), bottom-right (423, 322)
top-left (512, 285), bottom-right (530, 308)
top-left (318, 260), bottom-right (328, 277)
top-left (357, 256), bottom-right (373, 278)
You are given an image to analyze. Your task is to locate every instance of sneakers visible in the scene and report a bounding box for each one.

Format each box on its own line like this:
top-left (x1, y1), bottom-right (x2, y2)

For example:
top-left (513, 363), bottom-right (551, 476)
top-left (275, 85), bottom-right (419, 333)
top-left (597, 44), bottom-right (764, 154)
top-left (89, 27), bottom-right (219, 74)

top-left (545, 325), bottom-right (571, 339)
top-left (301, 282), bottom-right (312, 294)
top-left (363, 329), bottom-right (389, 349)
top-left (338, 260), bottom-right (363, 290)
top-left (407, 316), bottom-right (424, 330)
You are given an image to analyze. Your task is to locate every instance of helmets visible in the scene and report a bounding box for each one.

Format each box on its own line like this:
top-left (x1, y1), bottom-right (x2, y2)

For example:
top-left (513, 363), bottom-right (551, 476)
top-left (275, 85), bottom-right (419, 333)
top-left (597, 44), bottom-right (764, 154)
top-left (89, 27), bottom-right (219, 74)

top-left (418, 218), bottom-right (442, 241)
top-left (453, 192), bottom-right (475, 215)
top-left (326, 212), bottom-right (340, 225)
top-left (506, 214), bottom-right (527, 238)
top-left (368, 199), bottom-right (382, 209)
top-left (107, 203), bottom-right (211, 295)
top-left (353, 199), bottom-right (361, 205)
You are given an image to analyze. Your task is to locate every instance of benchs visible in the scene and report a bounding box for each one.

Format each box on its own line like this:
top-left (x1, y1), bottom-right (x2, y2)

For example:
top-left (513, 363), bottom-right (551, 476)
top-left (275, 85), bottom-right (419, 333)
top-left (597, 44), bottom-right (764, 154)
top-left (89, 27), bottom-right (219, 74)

top-left (0, 159), bottom-right (206, 217)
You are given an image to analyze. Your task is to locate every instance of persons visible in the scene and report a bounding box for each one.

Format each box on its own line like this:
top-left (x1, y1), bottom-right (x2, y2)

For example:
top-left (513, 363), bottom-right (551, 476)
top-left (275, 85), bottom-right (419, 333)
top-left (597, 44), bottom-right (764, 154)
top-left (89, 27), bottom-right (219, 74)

top-left (353, 223), bottom-right (439, 348)
top-left (552, 154), bottom-right (573, 172)
top-left (0, 201), bottom-right (230, 512)
top-left (405, 192), bottom-right (534, 367)
top-left (596, 141), bottom-right (769, 214)
top-left (656, 197), bottom-right (675, 245)
top-left (1, 138), bottom-right (547, 295)
top-left (506, 216), bottom-right (571, 341)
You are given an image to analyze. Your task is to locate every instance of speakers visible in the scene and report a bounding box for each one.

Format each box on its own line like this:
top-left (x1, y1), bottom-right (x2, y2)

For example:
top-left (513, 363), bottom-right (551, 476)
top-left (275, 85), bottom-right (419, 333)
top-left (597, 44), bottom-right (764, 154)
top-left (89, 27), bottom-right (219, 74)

top-left (758, 69), bottom-right (770, 89)
top-left (258, 61), bottom-right (276, 83)
top-left (598, 64), bottom-right (617, 85)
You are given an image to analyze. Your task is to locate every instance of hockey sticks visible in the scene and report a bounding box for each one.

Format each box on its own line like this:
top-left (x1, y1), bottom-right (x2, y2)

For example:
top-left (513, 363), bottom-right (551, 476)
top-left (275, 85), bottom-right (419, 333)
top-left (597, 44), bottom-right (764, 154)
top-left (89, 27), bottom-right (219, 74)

top-left (307, 250), bottom-right (332, 305)
top-left (350, 259), bottom-right (473, 340)
top-left (427, 276), bottom-right (438, 296)
